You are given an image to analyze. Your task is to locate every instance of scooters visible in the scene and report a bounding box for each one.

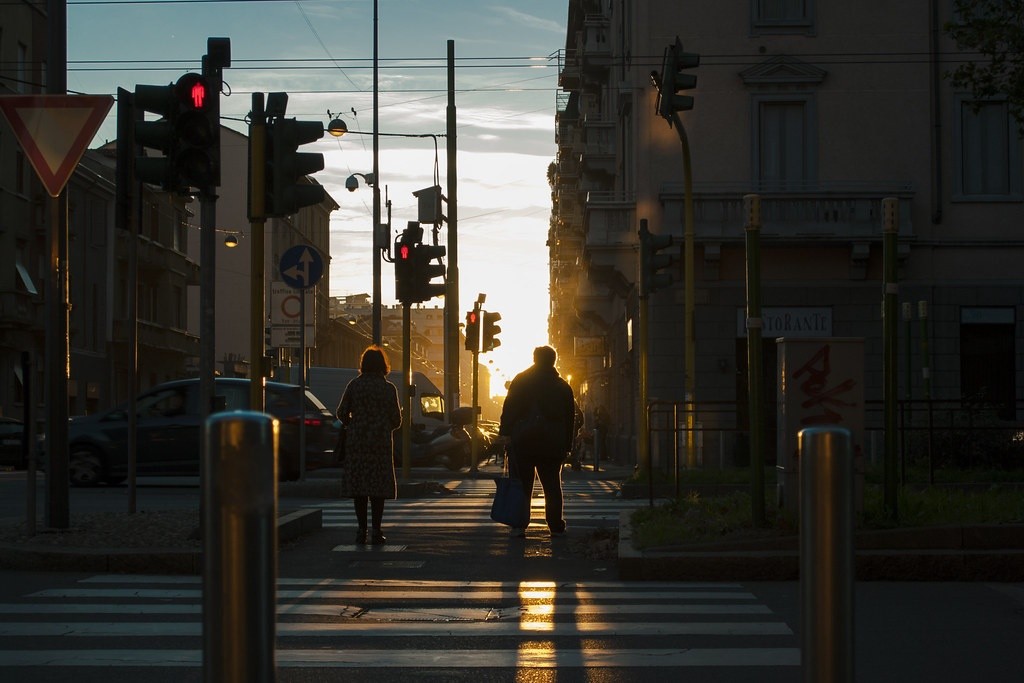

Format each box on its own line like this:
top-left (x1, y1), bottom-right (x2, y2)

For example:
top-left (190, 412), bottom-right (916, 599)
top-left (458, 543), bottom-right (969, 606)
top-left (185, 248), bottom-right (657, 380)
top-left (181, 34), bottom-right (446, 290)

top-left (392, 407), bottom-right (474, 472)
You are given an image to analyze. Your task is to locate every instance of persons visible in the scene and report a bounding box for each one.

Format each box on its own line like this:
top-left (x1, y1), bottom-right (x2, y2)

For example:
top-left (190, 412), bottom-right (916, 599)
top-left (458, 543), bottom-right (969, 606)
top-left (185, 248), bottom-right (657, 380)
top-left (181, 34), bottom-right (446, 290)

top-left (337, 349), bottom-right (403, 544)
top-left (499, 348), bottom-right (577, 536)
top-left (568, 403), bottom-right (610, 464)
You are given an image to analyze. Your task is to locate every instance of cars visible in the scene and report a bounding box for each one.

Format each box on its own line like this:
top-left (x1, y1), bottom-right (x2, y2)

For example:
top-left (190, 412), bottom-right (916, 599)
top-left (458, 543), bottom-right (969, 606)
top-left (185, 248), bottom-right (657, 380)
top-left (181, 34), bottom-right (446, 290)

top-left (0, 415), bottom-right (76, 471)
top-left (36, 376), bottom-right (341, 487)
top-left (465, 419), bottom-right (503, 460)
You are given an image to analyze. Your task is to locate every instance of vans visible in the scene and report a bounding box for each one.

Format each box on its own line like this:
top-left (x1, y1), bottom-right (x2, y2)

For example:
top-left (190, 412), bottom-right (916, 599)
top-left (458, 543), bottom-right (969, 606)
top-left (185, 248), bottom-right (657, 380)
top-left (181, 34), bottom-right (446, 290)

top-left (274, 364), bottom-right (447, 440)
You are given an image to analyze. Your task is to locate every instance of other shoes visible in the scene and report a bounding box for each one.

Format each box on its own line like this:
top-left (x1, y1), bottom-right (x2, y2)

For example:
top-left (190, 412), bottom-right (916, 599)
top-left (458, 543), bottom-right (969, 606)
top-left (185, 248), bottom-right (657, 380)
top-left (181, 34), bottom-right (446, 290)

top-left (508, 526), bottom-right (526, 539)
top-left (371, 533), bottom-right (392, 543)
top-left (548, 519), bottom-right (567, 537)
top-left (356, 528), bottom-right (368, 543)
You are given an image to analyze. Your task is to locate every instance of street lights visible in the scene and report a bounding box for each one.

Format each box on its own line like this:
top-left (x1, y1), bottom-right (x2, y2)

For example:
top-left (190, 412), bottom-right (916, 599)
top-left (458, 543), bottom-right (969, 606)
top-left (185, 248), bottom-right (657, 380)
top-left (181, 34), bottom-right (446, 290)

top-left (345, 172), bottom-right (384, 346)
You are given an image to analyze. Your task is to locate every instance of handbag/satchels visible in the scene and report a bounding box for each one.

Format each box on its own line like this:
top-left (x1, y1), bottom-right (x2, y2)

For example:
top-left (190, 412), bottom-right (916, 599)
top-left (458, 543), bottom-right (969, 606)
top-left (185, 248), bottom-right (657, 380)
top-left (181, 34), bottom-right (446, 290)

top-left (490, 442), bottom-right (531, 528)
top-left (333, 422), bottom-right (348, 462)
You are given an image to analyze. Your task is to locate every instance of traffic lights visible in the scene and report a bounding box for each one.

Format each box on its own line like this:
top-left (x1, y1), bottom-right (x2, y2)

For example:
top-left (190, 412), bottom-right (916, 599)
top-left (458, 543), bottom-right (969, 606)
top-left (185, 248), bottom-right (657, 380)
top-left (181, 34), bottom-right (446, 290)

top-left (394, 241), bottom-right (420, 305)
top-left (482, 311), bottom-right (501, 351)
top-left (658, 45), bottom-right (700, 120)
top-left (172, 72), bottom-right (213, 187)
top-left (268, 116), bottom-right (326, 218)
top-left (465, 311), bottom-right (479, 351)
top-left (638, 218), bottom-right (674, 300)
top-left (132, 80), bottom-right (179, 189)
top-left (414, 243), bottom-right (448, 303)
